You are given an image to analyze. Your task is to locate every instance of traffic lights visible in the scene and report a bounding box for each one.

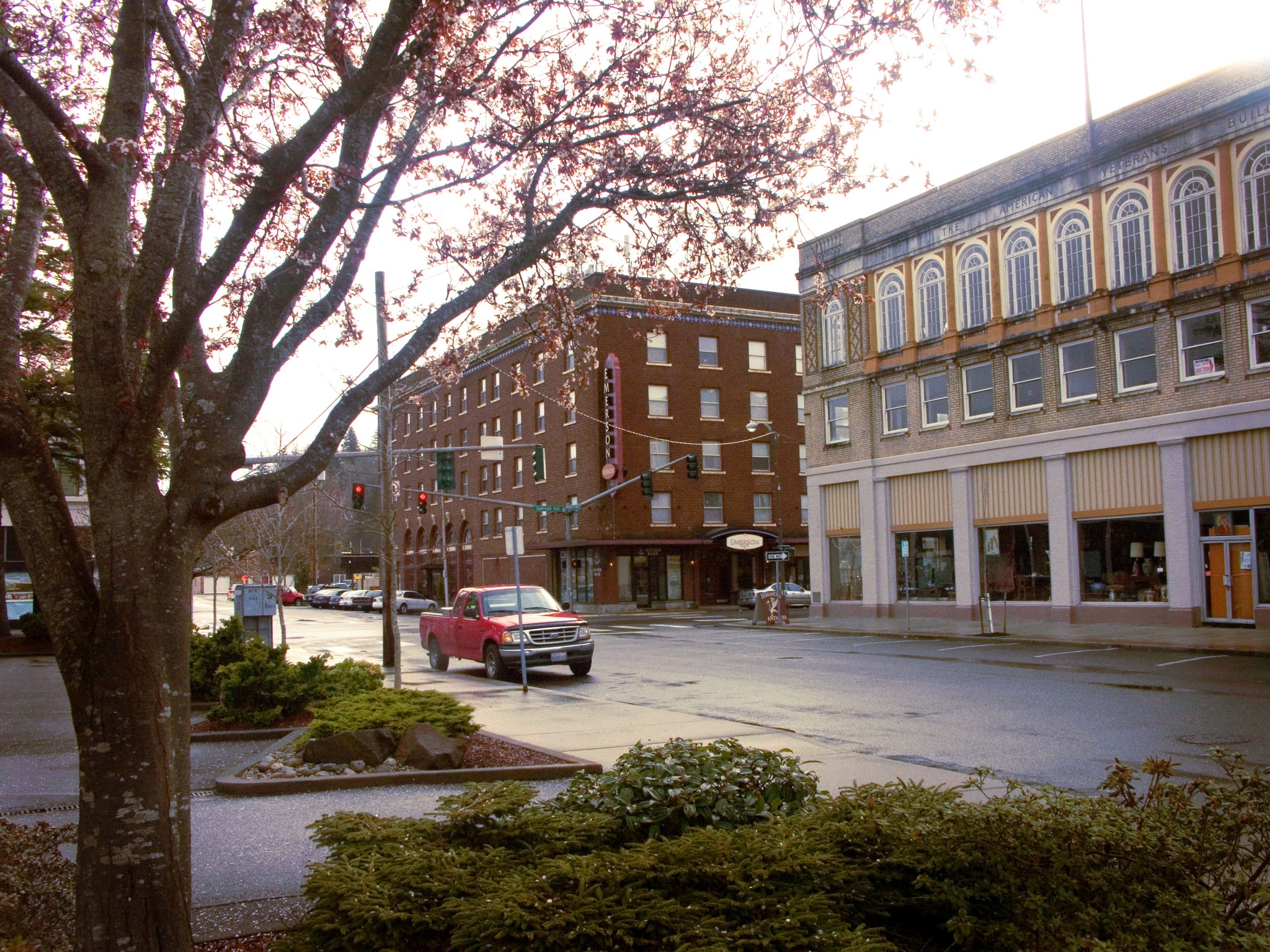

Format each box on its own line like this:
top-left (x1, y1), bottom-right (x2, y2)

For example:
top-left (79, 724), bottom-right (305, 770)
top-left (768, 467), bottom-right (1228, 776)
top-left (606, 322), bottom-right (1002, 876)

top-left (419, 492), bottom-right (427, 514)
top-left (687, 453), bottom-right (698, 479)
top-left (640, 470), bottom-right (653, 498)
top-left (532, 448), bottom-right (544, 480)
top-left (352, 483), bottom-right (365, 510)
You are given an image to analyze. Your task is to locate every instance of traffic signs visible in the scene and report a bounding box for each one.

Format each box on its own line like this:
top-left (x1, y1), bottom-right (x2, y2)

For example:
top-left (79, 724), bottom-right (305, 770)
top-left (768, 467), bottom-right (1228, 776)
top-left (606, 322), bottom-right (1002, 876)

top-left (765, 552), bottom-right (789, 561)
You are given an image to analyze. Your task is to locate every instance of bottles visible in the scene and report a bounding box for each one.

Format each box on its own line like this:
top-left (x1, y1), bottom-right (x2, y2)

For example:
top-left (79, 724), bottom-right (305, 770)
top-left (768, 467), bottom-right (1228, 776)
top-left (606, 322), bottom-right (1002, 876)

top-left (1109, 589), bottom-right (1116, 602)
top-left (1142, 559), bottom-right (1154, 576)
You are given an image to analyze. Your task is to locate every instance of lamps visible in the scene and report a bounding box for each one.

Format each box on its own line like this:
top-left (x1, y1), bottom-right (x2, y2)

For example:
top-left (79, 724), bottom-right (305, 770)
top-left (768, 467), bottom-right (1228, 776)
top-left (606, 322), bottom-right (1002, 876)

top-left (1154, 541), bottom-right (1166, 568)
top-left (1131, 542), bottom-right (1144, 576)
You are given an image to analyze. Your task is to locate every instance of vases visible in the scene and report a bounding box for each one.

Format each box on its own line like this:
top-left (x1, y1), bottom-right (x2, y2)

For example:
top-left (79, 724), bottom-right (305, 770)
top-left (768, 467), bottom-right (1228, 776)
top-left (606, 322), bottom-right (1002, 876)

top-left (1161, 585), bottom-right (1168, 599)
top-left (1142, 559), bottom-right (1154, 576)
top-left (1109, 589), bottom-right (1115, 602)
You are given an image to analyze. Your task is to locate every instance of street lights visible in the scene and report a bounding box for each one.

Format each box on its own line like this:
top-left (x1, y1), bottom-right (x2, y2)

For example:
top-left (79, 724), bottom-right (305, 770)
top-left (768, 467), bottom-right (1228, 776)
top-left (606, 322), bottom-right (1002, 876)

top-left (747, 421), bottom-right (786, 604)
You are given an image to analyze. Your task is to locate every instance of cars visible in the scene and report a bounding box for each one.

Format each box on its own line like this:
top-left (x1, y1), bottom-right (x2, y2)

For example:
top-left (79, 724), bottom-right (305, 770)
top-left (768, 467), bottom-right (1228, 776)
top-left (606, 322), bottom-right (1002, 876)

top-left (352, 591), bottom-right (382, 613)
top-left (227, 584), bottom-right (240, 600)
top-left (302, 579), bottom-right (370, 611)
top-left (737, 583), bottom-right (811, 610)
top-left (276, 586), bottom-right (305, 607)
top-left (372, 591), bottom-right (438, 616)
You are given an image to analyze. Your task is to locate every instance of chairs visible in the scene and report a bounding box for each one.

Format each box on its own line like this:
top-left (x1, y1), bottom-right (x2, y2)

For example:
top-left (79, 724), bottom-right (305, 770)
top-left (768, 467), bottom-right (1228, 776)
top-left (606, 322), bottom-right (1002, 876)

top-left (515, 597), bottom-right (533, 608)
top-left (1016, 574), bottom-right (1036, 601)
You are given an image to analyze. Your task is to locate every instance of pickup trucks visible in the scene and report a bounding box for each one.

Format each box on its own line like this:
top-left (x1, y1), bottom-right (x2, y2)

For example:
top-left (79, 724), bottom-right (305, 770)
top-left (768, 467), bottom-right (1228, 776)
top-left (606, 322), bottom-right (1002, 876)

top-left (418, 586), bottom-right (594, 680)
